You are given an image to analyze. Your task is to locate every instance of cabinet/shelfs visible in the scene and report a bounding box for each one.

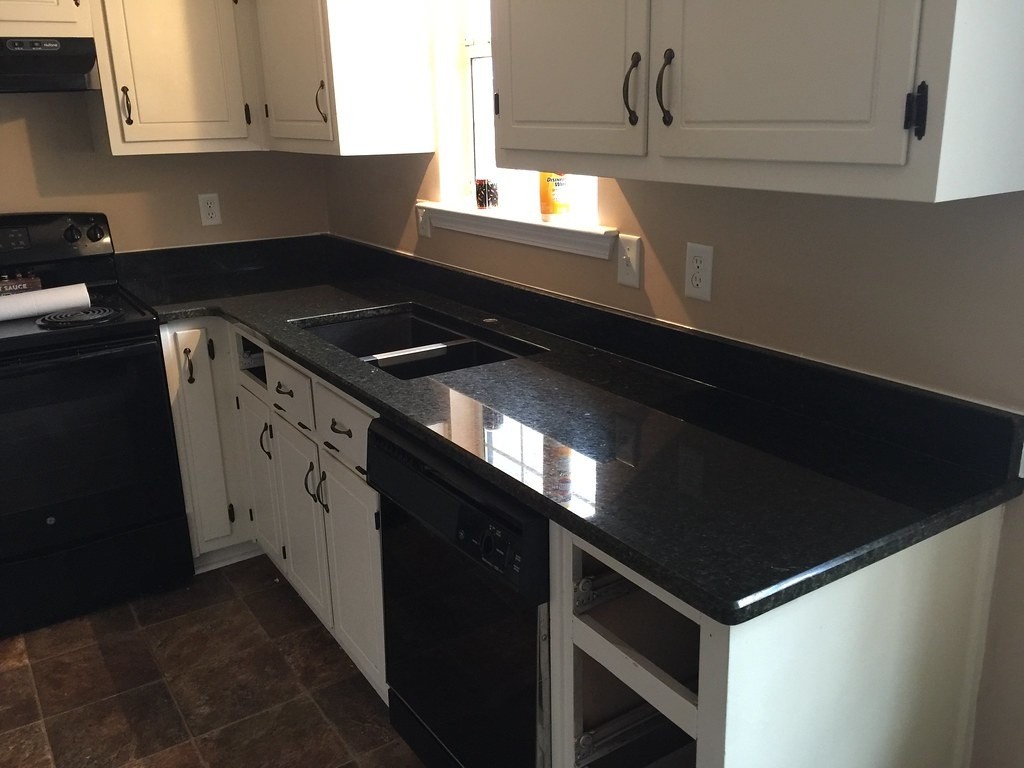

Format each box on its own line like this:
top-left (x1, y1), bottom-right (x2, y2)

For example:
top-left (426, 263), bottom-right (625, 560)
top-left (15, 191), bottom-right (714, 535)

top-left (87, 0), bottom-right (271, 156)
top-left (0, 0), bottom-right (94, 39)
top-left (490, 1), bottom-right (1024, 205)
top-left (159, 317), bottom-right (263, 576)
top-left (256, 0), bottom-right (436, 156)
top-left (550, 503), bottom-right (1006, 767)
top-left (228, 322), bottom-right (286, 579)
top-left (264, 346), bottom-right (389, 711)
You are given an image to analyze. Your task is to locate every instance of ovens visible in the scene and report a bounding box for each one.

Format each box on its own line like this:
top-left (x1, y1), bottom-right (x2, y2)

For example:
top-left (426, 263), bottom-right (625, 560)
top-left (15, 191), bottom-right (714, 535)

top-left (0, 335), bottom-right (196, 639)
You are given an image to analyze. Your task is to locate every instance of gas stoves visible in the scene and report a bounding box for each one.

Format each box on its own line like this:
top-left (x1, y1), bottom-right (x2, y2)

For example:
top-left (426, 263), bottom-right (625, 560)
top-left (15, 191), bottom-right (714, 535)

top-left (0, 212), bottom-right (158, 354)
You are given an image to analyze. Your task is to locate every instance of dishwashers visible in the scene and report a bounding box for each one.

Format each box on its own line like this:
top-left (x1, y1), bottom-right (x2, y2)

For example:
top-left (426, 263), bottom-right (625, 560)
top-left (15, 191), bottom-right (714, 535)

top-left (365, 420), bottom-right (538, 768)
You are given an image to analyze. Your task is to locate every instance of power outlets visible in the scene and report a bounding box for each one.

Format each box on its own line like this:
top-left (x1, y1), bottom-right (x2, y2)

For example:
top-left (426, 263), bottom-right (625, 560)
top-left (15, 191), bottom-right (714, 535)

top-left (685, 242), bottom-right (714, 303)
top-left (197, 193), bottom-right (222, 226)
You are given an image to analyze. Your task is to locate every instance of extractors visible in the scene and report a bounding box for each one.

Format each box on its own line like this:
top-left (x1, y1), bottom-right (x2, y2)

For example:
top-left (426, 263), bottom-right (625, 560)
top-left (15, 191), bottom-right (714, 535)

top-left (0, 37), bottom-right (96, 92)
